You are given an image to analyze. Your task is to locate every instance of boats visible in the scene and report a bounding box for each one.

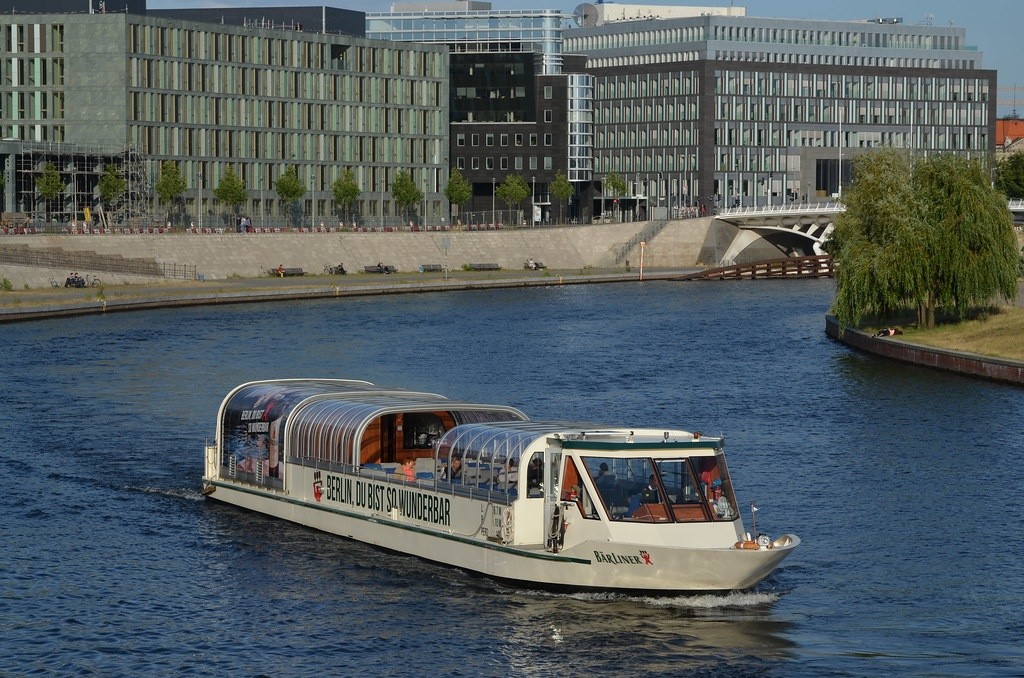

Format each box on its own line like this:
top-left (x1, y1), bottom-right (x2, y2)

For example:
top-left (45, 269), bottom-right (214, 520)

top-left (201, 376), bottom-right (802, 595)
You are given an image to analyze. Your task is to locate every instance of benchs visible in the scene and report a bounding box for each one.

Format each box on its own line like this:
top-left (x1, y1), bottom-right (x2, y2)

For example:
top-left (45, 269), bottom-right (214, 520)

top-left (330, 267), bottom-right (347, 274)
top-left (422, 265), bottom-right (444, 272)
top-left (523, 263), bottom-right (547, 270)
top-left (271, 268), bottom-right (307, 277)
top-left (468, 264), bottom-right (501, 271)
top-left (364, 266), bottom-right (398, 274)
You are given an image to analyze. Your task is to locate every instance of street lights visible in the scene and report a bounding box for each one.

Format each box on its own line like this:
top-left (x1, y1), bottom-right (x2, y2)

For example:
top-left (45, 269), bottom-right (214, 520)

top-left (532, 176), bottom-right (536, 228)
top-left (807, 181), bottom-right (812, 209)
top-left (601, 177), bottom-right (607, 223)
top-left (491, 177), bottom-right (498, 229)
top-left (260, 175), bottom-right (265, 230)
top-left (197, 172), bottom-right (203, 234)
top-left (311, 174), bottom-right (316, 233)
top-left (423, 177), bottom-right (428, 233)
top-left (380, 177), bottom-right (384, 230)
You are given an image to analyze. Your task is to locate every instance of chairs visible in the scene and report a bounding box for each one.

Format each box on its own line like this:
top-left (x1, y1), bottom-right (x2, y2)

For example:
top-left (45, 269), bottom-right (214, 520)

top-left (363, 457), bottom-right (544, 503)
top-left (627, 495), bottom-right (641, 518)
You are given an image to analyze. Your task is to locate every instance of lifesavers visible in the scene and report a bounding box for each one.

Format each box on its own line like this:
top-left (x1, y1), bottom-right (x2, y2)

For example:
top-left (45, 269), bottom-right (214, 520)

top-left (736, 540), bottom-right (759, 550)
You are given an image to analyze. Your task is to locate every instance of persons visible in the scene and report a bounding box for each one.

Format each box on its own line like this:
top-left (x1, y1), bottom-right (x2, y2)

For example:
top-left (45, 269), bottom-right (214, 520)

top-left (643, 474), bottom-right (658, 502)
top-left (526, 257), bottom-right (535, 269)
top-left (700, 203), bottom-right (705, 215)
top-left (623, 491), bottom-right (641, 517)
top-left (236, 215), bottom-right (251, 231)
top-left (393, 456), bottom-right (416, 482)
top-left (595, 462), bottom-right (617, 489)
top-left (528, 458), bottom-right (542, 481)
top-left (873, 326), bottom-right (902, 335)
top-left (457, 217), bottom-right (462, 225)
top-left (68, 272), bottom-right (79, 285)
top-left (377, 261), bottom-right (389, 274)
top-left (497, 457), bottom-right (514, 484)
top-left (443, 457), bottom-right (463, 478)
top-left (278, 263), bottom-right (284, 276)
